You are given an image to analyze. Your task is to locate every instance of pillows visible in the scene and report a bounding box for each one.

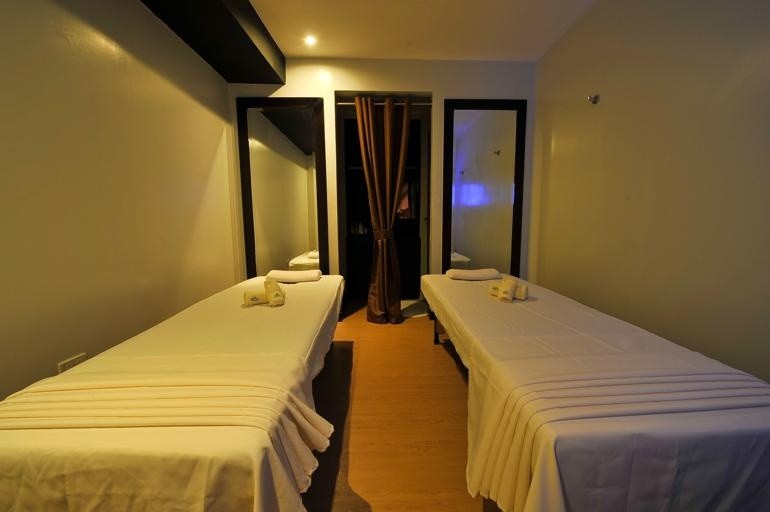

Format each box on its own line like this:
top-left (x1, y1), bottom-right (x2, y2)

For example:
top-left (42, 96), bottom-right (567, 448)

top-left (264, 269), bottom-right (322, 283)
top-left (444, 267), bottom-right (501, 280)
top-left (308, 250), bottom-right (319, 259)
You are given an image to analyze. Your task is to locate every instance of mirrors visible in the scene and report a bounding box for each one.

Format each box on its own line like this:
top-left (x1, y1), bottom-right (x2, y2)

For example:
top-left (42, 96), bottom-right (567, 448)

top-left (235, 94), bottom-right (328, 279)
top-left (442, 99), bottom-right (526, 274)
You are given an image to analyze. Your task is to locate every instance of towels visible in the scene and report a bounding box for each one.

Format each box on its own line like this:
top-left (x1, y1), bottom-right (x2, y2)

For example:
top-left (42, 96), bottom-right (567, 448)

top-left (242, 287), bottom-right (267, 306)
top-left (488, 278), bottom-right (529, 301)
top-left (497, 275), bottom-right (519, 302)
top-left (263, 279), bottom-right (285, 306)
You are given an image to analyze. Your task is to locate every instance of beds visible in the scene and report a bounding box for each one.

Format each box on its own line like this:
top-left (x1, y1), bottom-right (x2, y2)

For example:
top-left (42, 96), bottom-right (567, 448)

top-left (1, 270), bottom-right (349, 510)
top-left (449, 251), bottom-right (472, 267)
top-left (418, 269), bottom-right (770, 512)
top-left (288, 250), bottom-right (320, 269)
